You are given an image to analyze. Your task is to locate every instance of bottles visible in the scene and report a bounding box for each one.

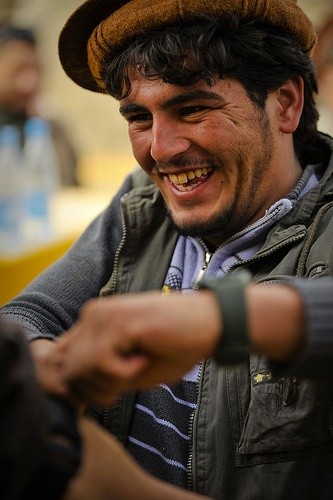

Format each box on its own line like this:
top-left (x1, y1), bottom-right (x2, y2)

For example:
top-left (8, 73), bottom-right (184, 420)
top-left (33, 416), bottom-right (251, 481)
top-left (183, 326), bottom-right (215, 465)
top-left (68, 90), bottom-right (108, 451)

top-left (2, 117), bottom-right (59, 262)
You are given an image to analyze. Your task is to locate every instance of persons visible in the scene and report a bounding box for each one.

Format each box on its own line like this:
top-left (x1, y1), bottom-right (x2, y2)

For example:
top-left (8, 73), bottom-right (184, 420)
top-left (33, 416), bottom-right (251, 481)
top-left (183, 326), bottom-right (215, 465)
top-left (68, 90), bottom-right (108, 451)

top-left (0, 2), bottom-right (333, 497)
top-left (0, 309), bottom-right (217, 500)
top-left (0, 21), bottom-right (81, 263)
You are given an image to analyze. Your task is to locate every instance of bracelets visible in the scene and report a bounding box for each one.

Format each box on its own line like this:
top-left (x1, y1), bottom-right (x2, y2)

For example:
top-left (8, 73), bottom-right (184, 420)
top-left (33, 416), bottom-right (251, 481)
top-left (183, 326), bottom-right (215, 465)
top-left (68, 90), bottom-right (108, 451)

top-left (197, 277), bottom-right (251, 368)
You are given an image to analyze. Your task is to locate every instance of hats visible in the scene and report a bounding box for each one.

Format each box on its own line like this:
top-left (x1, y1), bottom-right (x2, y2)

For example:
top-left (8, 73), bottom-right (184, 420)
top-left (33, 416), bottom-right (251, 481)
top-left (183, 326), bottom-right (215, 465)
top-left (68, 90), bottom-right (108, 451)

top-left (58, 0), bottom-right (317, 95)
